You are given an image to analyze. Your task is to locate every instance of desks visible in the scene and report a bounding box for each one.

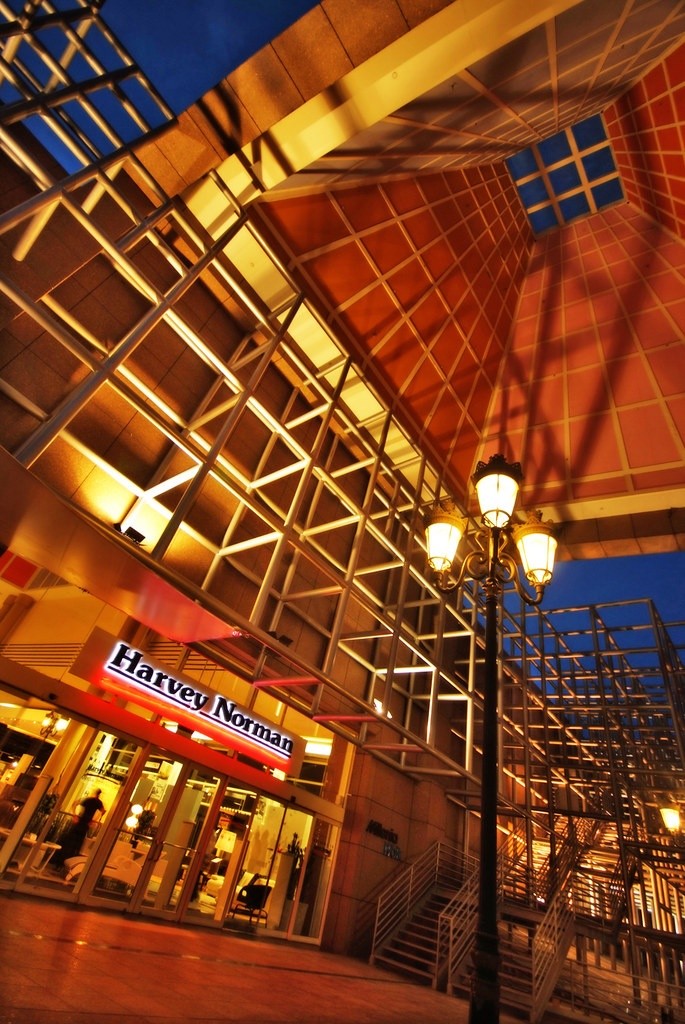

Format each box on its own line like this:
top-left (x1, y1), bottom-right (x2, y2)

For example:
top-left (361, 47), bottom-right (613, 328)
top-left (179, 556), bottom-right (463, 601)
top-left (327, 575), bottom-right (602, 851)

top-left (0, 827), bottom-right (61, 873)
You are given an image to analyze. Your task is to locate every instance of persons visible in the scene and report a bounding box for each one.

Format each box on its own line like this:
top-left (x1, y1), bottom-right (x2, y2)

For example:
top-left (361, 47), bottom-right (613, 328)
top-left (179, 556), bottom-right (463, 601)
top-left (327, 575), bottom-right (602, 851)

top-left (62, 787), bottom-right (105, 856)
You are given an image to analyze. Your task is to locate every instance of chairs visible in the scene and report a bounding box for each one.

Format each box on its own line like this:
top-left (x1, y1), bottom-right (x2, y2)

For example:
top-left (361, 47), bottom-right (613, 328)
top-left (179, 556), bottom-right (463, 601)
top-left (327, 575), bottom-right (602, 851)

top-left (64, 850), bottom-right (167, 897)
top-left (230, 884), bottom-right (271, 928)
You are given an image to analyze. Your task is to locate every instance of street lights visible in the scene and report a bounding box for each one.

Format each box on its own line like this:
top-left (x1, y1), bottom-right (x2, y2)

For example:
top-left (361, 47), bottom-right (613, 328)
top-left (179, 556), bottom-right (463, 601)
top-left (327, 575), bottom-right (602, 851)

top-left (424, 455), bottom-right (560, 1024)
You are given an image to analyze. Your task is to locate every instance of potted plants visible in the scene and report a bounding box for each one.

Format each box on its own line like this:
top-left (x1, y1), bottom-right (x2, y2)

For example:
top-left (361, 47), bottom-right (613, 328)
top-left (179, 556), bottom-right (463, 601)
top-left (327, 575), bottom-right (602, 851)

top-left (25, 795), bottom-right (59, 840)
top-left (130, 810), bottom-right (157, 848)
top-left (285, 833), bottom-right (304, 900)
